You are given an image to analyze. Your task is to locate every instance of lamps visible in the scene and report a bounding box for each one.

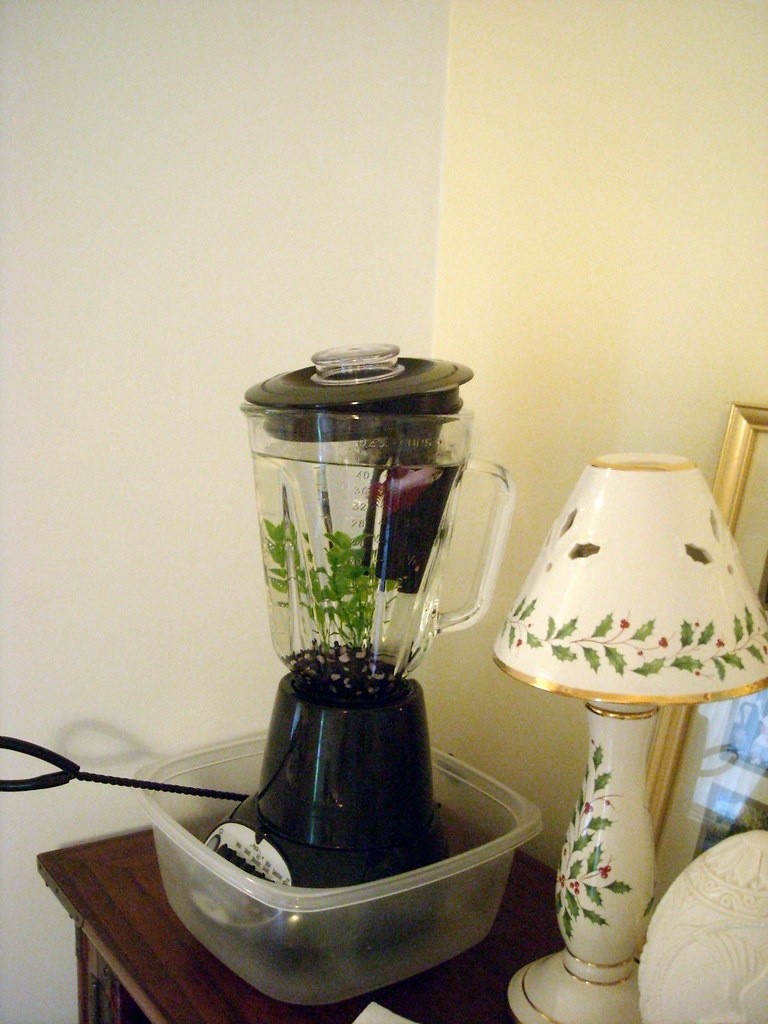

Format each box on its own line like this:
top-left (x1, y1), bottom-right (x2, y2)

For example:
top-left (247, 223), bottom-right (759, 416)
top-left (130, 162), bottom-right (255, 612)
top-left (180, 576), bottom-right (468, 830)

top-left (492, 451), bottom-right (768, 1024)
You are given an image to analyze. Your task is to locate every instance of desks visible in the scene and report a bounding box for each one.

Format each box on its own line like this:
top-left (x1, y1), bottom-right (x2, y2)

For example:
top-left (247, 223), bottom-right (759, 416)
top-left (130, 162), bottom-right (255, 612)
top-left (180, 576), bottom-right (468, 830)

top-left (35, 799), bottom-right (565, 1024)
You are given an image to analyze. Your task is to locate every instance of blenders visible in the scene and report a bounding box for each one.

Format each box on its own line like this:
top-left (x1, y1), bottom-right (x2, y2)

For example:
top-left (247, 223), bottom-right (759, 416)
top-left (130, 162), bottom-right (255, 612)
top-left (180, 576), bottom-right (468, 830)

top-left (186, 336), bottom-right (515, 956)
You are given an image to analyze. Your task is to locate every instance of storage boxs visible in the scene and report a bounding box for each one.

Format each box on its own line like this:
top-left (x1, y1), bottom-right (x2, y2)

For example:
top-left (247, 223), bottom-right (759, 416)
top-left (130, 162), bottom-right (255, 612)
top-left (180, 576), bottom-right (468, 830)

top-left (132, 730), bottom-right (544, 1004)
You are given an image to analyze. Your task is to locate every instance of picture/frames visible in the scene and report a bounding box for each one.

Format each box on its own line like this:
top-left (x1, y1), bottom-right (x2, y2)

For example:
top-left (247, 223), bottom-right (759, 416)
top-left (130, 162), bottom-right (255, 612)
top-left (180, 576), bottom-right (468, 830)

top-left (635, 402), bottom-right (768, 957)
